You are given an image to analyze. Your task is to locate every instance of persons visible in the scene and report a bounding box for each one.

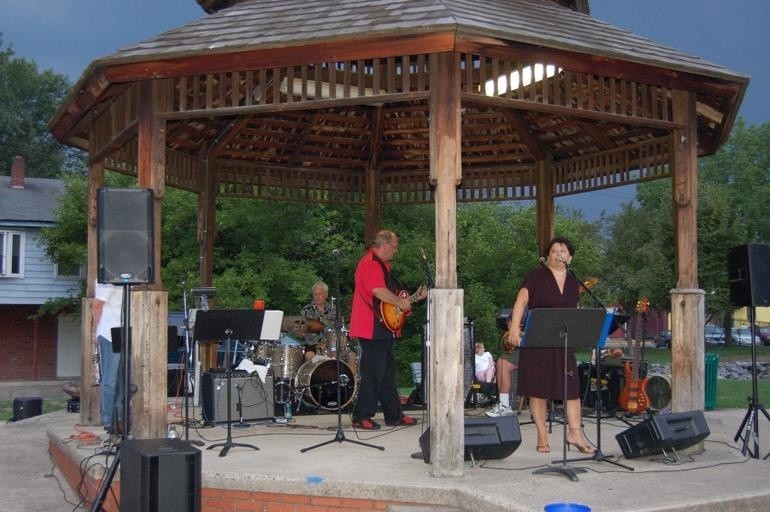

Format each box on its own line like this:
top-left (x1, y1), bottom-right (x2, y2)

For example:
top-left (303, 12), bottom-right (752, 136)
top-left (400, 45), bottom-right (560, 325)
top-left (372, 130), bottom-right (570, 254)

top-left (300, 281), bottom-right (357, 400)
top-left (349, 230), bottom-right (428, 429)
top-left (92, 278), bottom-right (131, 434)
top-left (486, 306), bottom-right (528, 417)
top-left (578, 303), bottom-right (627, 417)
top-left (509, 237), bottom-right (597, 453)
top-left (475, 342), bottom-right (499, 408)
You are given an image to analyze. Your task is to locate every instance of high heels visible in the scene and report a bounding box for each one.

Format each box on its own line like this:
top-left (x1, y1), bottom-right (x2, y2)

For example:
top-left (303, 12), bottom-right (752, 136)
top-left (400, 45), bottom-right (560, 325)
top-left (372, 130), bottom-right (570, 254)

top-left (536, 444), bottom-right (550, 452)
top-left (566, 439), bottom-right (595, 453)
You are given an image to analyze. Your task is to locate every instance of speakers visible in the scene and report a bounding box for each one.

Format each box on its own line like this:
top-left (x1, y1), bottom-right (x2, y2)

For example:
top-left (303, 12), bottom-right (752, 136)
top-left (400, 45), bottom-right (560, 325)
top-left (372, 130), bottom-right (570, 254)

top-left (419, 415), bottom-right (522, 463)
top-left (98, 188), bottom-right (154, 285)
top-left (726, 243), bottom-right (770, 307)
top-left (616, 410), bottom-right (709, 458)
top-left (118, 438), bottom-right (202, 512)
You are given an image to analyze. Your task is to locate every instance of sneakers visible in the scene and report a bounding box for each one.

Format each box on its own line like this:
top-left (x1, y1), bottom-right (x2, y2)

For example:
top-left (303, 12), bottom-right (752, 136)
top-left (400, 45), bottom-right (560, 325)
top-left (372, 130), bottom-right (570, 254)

top-left (485, 401), bottom-right (512, 418)
top-left (104, 425), bottom-right (118, 435)
top-left (352, 418), bottom-right (381, 430)
top-left (398, 415), bottom-right (417, 425)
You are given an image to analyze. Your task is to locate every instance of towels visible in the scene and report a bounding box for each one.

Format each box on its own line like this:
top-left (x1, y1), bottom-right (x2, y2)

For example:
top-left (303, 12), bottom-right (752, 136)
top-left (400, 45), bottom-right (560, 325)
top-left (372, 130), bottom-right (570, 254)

top-left (235, 359), bottom-right (270, 384)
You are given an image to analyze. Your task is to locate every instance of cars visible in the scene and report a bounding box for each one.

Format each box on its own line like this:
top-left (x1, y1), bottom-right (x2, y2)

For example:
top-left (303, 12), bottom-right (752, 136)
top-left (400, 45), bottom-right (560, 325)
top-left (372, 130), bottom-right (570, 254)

top-left (704, 325), bottom-right (725, 345)
top-left (653, 329), bottom-right (672, 348)
top-left (731, 327), bottom-right (760, 346)
top-left (754, 322), bottom-right (769, 346)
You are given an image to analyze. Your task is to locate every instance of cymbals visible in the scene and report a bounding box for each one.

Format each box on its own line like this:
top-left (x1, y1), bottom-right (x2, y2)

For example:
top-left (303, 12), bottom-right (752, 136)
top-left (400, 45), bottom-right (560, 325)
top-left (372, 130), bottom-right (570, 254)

top-left (280, 316), bottom-right (325, 334)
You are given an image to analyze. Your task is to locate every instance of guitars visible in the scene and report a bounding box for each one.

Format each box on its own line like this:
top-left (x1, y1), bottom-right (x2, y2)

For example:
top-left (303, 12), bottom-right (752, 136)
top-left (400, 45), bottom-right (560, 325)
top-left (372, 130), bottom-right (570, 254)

top-left (380, 285), bottom-right (429, 333)
top-left (503, 276), bottom-right (598, 353)
top-left (618, 297), bottom-right (650, 414)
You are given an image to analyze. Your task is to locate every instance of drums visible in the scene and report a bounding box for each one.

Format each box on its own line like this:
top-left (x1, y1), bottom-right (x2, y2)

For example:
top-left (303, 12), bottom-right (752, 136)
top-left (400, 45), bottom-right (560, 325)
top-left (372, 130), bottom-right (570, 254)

top-left (294, 357), bottom-right (357, 410)
top-left (253, 345), bottom-right (272, 360)
top-left (325, 328), bottom-right (347, 352)
top-left (271, 346), bottom-right (303, 378)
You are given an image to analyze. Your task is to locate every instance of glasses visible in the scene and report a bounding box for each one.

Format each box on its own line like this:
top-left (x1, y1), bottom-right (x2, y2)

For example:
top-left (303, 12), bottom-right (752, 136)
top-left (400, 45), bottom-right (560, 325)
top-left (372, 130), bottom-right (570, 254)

top-left (480, 347), bottom-right (485, 349)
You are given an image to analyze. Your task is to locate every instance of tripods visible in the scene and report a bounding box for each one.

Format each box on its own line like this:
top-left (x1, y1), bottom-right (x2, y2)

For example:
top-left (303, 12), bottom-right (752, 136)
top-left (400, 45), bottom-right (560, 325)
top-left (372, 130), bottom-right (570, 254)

top-left (523, 308), bottom-right (606, 484)
top-left (300, 267), bottom-right (385, 454)
top-left (194, 310), bottom-right (265, 457)
top-left (551, 270), bottom-right (634, 471)
top-left (734, 307), bottom-right (770, 460)
top-left (90, 288), bottom-right (132, 511)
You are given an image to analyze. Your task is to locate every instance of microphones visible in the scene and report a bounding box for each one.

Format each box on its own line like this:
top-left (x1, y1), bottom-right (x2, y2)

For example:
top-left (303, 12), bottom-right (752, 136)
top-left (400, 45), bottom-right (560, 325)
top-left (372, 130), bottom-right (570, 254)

top-left (418, 247), bottom-right (428, 263)
top-left (331, 248), bottom-right (340, 264)
top-left (555, 255), bottom-right (569, 267)
